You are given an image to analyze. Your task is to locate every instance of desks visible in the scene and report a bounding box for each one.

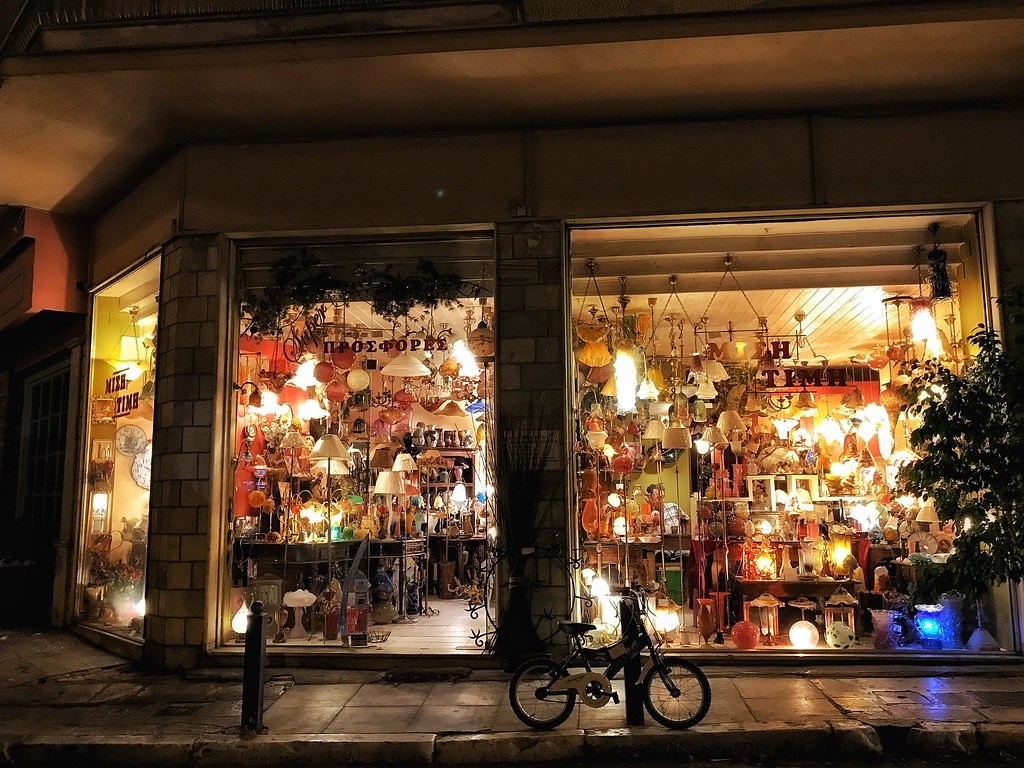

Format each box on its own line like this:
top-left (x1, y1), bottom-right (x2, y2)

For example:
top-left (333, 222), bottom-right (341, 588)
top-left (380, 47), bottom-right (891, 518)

top-left (240, 541), bottom-right (357, 630)
top-left (721, 572), bottom-right (861, 641)
top-left (584, 542), bottom-right (656, 588)
top-left (347, 537), bottom-right (426, 616)
top-left (657, 534), bottom-right (692, 551)
top-left (690, 539), bottom-right (861, 633)
top-left (864, 544), bottom-right (908, 632)
top-left (442, 538), bottom-right (486, 587)
top-left (890, 560), bottom-right (953, 598)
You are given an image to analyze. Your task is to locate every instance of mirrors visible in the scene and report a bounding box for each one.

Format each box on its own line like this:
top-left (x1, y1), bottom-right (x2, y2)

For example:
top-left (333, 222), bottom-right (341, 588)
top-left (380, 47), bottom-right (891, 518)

top-left (78, 476), bottom-right (112, 613)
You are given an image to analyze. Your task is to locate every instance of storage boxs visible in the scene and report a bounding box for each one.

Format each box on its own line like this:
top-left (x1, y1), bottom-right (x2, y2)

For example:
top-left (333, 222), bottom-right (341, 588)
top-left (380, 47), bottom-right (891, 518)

top-left (665, 591), bottom-right (682, 606)
top-left (655, 569), bottom-right (684, 590)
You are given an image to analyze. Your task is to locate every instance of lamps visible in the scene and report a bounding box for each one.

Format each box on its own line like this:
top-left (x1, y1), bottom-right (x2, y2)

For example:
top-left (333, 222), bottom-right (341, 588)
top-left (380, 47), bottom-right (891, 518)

top-left (105, 222), bottom-right (1000, 651)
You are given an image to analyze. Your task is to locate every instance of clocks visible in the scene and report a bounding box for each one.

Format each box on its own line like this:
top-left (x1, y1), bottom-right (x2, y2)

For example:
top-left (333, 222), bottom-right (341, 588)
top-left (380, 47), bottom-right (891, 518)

top-left (255, 574), bottom-right (283, 608)
top-left (130, 439), bottom-right (152, 490)
top-left (114, 425), bottom-right (148, 457)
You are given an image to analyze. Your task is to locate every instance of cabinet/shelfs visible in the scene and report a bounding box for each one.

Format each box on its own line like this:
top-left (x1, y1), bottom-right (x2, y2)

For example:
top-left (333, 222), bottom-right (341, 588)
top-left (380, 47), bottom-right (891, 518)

top-left (411, 446), bottom-right (477, 535)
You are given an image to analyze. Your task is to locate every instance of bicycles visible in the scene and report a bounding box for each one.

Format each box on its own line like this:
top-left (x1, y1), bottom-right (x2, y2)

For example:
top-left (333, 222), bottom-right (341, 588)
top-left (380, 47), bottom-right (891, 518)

top-left (510, 584), bottom-right (711, 732)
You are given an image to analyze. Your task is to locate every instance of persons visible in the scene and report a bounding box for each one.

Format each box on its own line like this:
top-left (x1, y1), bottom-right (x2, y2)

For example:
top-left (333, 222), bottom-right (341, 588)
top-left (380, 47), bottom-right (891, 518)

top-left (646, 484), bottom-right (689, 571)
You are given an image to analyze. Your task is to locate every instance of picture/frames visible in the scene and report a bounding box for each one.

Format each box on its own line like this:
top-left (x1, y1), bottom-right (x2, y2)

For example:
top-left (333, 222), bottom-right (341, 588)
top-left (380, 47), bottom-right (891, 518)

top-left (91, 437), bottom-right (115, 464)
top-left (91, 394), bottom-right (116, 426)
